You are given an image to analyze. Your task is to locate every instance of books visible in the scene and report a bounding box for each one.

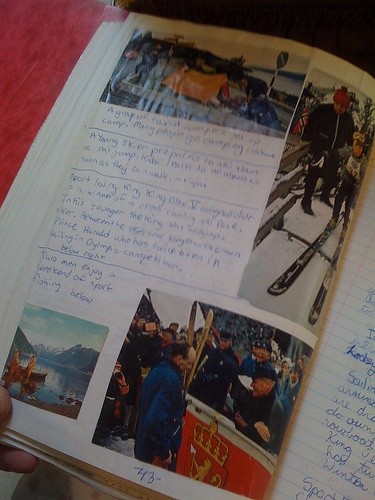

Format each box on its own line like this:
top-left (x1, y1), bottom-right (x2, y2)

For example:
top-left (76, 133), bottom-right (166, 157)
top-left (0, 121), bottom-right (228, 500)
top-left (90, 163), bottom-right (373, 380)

top-left (0, 11), bottom-right (375, 499)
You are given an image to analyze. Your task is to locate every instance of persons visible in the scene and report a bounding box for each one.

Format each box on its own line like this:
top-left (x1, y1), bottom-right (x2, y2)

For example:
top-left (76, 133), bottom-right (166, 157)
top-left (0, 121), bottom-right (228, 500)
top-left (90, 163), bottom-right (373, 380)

top-left (0, 385), bottom-right (40, 474)
top-left (300, 89), bottom-right (367, 231)
top-left (135, 41), bottom-right (278, 135)
top-left (94, 288), bottom-right (306, 485)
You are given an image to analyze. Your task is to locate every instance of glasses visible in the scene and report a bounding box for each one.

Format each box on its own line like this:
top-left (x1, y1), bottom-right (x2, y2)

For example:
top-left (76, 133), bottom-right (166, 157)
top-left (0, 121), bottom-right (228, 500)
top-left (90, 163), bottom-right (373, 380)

top-left (353, 133), bottom-right (365, 143)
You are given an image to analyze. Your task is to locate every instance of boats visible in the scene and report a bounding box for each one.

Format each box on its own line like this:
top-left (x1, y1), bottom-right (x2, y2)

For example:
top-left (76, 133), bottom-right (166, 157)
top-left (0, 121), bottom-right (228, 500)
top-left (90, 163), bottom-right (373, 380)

top-left (7, 363), bottom-right (48, 381)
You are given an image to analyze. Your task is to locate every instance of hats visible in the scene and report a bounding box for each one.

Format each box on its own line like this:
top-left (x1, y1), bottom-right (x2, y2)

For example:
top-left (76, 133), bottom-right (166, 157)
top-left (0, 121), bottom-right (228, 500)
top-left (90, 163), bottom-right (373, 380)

top-left (248, 365), bottom-right (277, 381)
top-left (161, 328), bottom-right (174, 335)
top-left (280, 358), bottom-right (292, 372)
top-left (334, 90), bottom-right (350, 108)
top-left (258, 342), bottom-right (272, 353)
top-left (220, 332), bottom-right (231, 340)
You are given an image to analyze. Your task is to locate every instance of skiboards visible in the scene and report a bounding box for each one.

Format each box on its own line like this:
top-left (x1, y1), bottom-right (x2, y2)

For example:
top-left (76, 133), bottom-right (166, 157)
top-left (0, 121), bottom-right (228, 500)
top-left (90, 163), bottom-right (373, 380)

top-left (267, 211), bottom-right (351, 327)
top-left (181, 299), bottom-right (215, 399)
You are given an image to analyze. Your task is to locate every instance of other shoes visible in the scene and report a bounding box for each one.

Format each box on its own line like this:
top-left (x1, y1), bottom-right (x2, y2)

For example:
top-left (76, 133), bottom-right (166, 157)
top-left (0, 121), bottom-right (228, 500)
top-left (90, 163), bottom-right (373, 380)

top-left (301, 197), bottom-right (314, 215)
top-left (320, 193), bottom-right (333, 207)
